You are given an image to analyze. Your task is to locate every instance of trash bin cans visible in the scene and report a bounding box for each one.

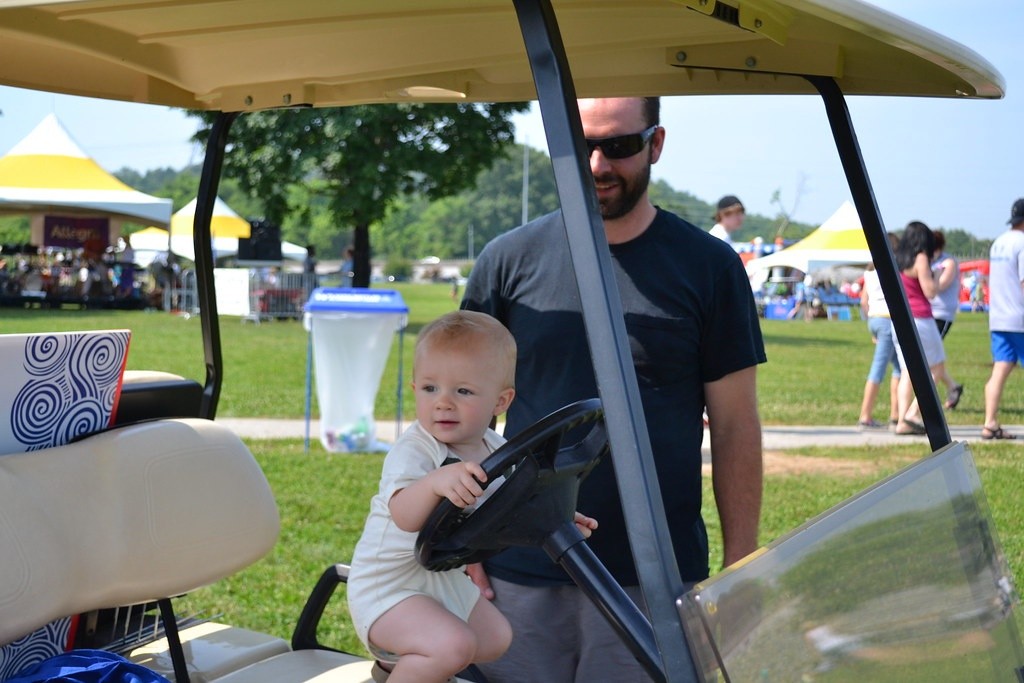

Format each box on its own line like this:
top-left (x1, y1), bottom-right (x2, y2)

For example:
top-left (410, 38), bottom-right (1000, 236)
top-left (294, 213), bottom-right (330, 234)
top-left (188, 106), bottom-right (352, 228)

top-left (302, 285), bottom-right (412, 455)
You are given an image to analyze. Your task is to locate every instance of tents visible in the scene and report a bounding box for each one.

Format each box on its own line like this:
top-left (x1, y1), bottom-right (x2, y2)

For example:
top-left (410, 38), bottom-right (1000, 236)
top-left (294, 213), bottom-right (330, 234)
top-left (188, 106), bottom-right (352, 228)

top-left (755, 203), bottom-right (873, 266)
top-left (142, 194), bottom-right (251, 238)
top-left (0, 114), bottom-right (174, 289)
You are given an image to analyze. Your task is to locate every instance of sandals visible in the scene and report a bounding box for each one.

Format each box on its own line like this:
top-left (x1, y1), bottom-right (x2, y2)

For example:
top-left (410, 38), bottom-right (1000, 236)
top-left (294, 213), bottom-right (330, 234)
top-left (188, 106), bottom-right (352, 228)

top-left (982, 426), bottom-right (1017, 439)
top-left (856, 419), bottom-right (884, 429)
top-left (896, 420), bottom-right (926, 435)
top-left (888, 420), bottom-right (899, 433)
top-left (945, 384), bottom-right (963, 409)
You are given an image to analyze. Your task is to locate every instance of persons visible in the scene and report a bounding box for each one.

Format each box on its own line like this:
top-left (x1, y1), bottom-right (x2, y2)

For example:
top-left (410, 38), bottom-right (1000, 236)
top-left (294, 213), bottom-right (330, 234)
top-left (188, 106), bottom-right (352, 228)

top-left (2, 230), bottom-right (141, 298)
top-left (340, 249), bottom-right (354, 289)
top-left (980, 198), bottom-right (1024, 439)
top-left (461, 95), bottom-right (767, 682)
top-left (708, 196), bottom-right (746, 246)
top-left (967, 270), bottom-right (984, 313)
top-left (765, 281), bottom-right (796, 321)
top-left (796, 275), bottom-right (819, 302)
top-left (925, 231), bottom-right (963, 410)
top-left (858, 231), bottom-right (901, 428)
top-left (753, 235), bottom-right (767, 258)
top-left (891, 221), bottom-right (942, 435)
top-left (347, 310), bottom-right (598, 683)
top-left (303, 246), bottom-right (320, 290)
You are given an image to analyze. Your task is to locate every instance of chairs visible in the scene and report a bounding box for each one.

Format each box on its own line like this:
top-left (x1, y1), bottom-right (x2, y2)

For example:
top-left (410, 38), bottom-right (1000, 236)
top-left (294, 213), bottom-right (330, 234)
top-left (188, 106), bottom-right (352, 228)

top-left (0, 415), bottom-right (377, 683)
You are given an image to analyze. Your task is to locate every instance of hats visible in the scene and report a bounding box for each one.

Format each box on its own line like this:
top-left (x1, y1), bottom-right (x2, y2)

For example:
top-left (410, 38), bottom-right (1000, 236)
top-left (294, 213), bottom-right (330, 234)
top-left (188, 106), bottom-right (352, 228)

top-left (718, 196), bottom-right (740, 208)
top-left (1005, 199), bottom-right (1024, 225)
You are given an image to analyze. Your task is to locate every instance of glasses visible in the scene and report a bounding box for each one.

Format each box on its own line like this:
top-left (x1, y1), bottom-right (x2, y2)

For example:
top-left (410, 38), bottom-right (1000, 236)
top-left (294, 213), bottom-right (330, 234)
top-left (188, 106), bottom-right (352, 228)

top-left (586, 125), bottom-right (658, 160)
